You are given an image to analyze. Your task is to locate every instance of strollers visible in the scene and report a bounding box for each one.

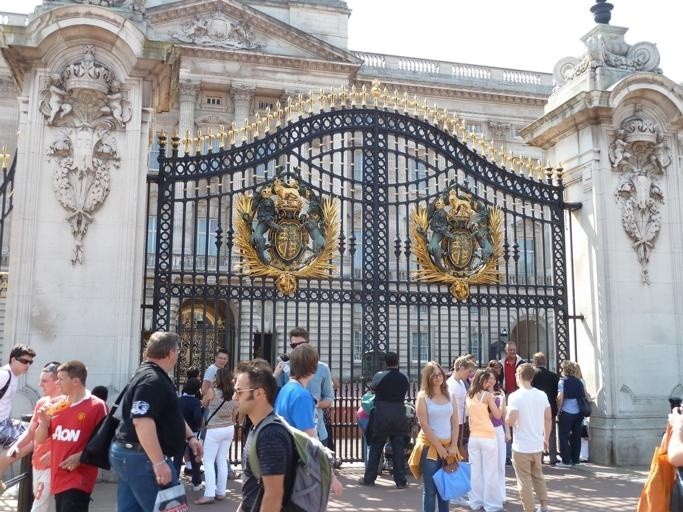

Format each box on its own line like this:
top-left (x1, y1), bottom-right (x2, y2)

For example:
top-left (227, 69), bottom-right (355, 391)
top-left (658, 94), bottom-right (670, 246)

top-left (383, 401), bottom-right (419, 474)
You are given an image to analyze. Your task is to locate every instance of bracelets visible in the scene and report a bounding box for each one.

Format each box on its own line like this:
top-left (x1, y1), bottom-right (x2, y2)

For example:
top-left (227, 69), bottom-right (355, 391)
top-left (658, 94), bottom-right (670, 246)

top-left (151, 460), bottom-right (165, 467)
top-left (185, 435), bottom-right (196, 442)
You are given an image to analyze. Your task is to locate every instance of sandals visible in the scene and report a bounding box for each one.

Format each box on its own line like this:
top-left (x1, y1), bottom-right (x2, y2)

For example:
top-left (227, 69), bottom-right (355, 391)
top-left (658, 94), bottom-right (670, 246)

top-left (193, 496), bottom-right (215, 505)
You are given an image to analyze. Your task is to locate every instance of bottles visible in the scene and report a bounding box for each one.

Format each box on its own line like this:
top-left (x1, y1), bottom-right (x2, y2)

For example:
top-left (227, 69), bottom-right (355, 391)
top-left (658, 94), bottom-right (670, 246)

top-left (41, 394), bottom-right (71, 414)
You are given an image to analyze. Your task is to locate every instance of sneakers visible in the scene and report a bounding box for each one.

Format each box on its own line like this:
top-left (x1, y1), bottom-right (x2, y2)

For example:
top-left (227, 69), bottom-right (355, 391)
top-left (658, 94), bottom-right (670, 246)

top-left (396, 482), bottom-right (409, 489)
top-left (359, 478), bottom-right (375, 486)
top-left (184, 465), bottom-right (205, 475)
top-left (193, 484), bottom-right (203, 492)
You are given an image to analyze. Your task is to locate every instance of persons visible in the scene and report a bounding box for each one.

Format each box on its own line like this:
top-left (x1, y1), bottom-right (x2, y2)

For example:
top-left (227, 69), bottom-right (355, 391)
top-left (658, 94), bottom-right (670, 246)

top-left (415, 341), bottom-right (587, 512)
top-left (43, 73), bottom-right (72, 125)
top-left (107, 332), bottom-right (204, 511)
top-left (646, 131), bottom-right (666, 176)
top-left (359, 351), bottom-right (409, 489)
top-left (1, 344), bottom-right (110, 512)
top-left (610, 128), bottom-right (630, 169)
top-left (176, 343), bottom-right (342, 512)
top-left (271, 326), bottom-right (334, 449)
top-left (668, 407), bottom-right (682, 512)
top-left (98, 79), bottom-right (125, 129)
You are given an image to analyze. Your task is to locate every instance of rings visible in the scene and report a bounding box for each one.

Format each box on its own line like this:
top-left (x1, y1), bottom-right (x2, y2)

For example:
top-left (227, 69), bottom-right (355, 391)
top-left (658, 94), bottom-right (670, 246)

top-left (156, 476), bottom-right (160, 480)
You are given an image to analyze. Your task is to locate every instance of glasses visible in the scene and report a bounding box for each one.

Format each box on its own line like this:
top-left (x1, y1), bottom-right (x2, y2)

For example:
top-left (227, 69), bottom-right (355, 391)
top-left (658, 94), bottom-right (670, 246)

top-left (290, 342), bottom-right (305, 350)
top-left (16, 357), bottom-right (33, 365)
top-left (234, 387), bottom-right (255, 393)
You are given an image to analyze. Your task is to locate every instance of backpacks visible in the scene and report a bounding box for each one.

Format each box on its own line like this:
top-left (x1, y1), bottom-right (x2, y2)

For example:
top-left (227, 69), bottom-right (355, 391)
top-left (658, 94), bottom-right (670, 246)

top-left (248, 415), bottom-right (333, 512)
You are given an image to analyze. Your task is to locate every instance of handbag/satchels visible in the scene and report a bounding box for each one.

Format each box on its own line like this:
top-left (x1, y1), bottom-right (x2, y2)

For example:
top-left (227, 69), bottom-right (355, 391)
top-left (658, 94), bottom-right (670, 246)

top-left (79, 413), bottom-right (120, 471)
top-left (197, 426), bottom-right (207, 440)
top-left (578, 396), bottom-right (592, 417)
top-left (432, 461), bottom-right (472, 501)
top-left (636, 447), bottom-right (675, 512)
